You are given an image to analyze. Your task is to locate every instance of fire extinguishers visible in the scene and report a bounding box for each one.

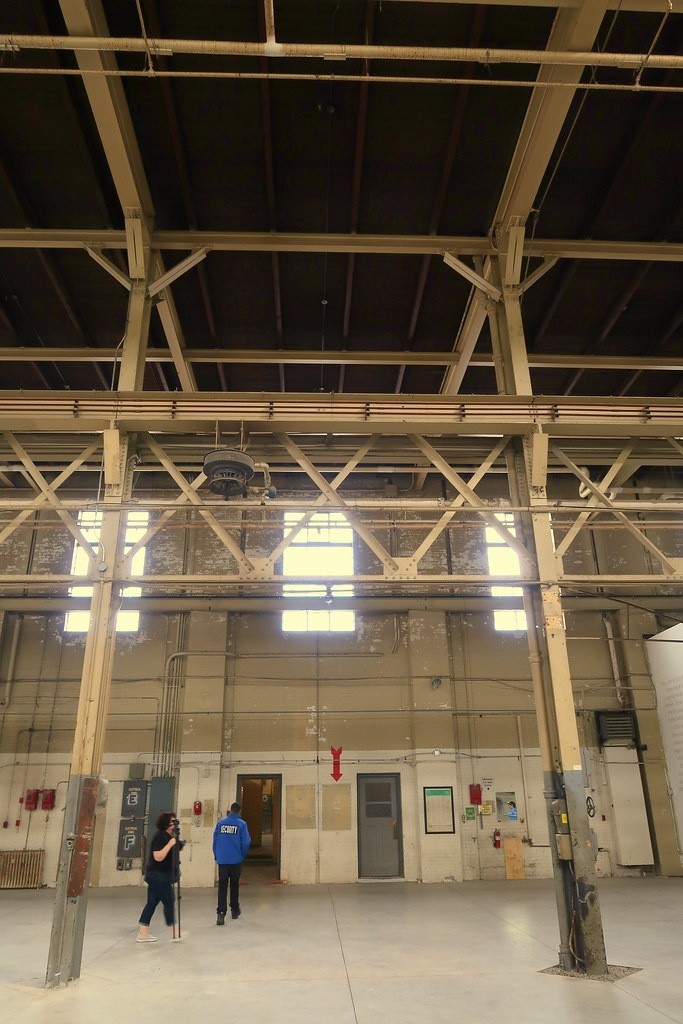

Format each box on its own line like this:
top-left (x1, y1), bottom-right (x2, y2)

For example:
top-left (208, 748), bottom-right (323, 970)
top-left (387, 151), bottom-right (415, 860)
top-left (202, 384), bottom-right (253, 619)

top-left (493, 829), bottom-right (500, 849)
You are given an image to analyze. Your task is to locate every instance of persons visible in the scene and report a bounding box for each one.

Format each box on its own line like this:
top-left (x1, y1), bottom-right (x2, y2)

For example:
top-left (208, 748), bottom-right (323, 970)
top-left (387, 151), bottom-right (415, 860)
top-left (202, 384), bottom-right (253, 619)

top-left (136, 812), bottom-right (188, 942)
top-left (213, 802), bottom-right (250, 924)
top-left (503, 801), bottom-right (517, 820)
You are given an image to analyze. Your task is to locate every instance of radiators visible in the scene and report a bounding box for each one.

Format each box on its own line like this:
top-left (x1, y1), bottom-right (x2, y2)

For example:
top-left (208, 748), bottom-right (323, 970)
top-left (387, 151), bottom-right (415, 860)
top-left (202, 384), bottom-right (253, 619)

top-left (0, 848), bottom-right (46, 890)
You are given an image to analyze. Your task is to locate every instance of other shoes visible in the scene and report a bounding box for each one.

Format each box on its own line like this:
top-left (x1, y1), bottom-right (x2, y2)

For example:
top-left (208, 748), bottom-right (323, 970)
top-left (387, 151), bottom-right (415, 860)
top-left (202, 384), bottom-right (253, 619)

top-left (137, 934), bottom-right (157, 942)
top-left (231, 908), bottom-right (241, 919)
top-left (169, 936), bottom-right (183, 942)
top-left (217, 911), bottom-right (226, 925)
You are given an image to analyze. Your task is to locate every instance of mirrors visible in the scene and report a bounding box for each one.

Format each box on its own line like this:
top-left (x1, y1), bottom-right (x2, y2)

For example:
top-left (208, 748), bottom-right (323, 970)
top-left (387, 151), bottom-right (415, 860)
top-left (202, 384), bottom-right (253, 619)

top-left (495, 792), bottom-right (518, 821)
